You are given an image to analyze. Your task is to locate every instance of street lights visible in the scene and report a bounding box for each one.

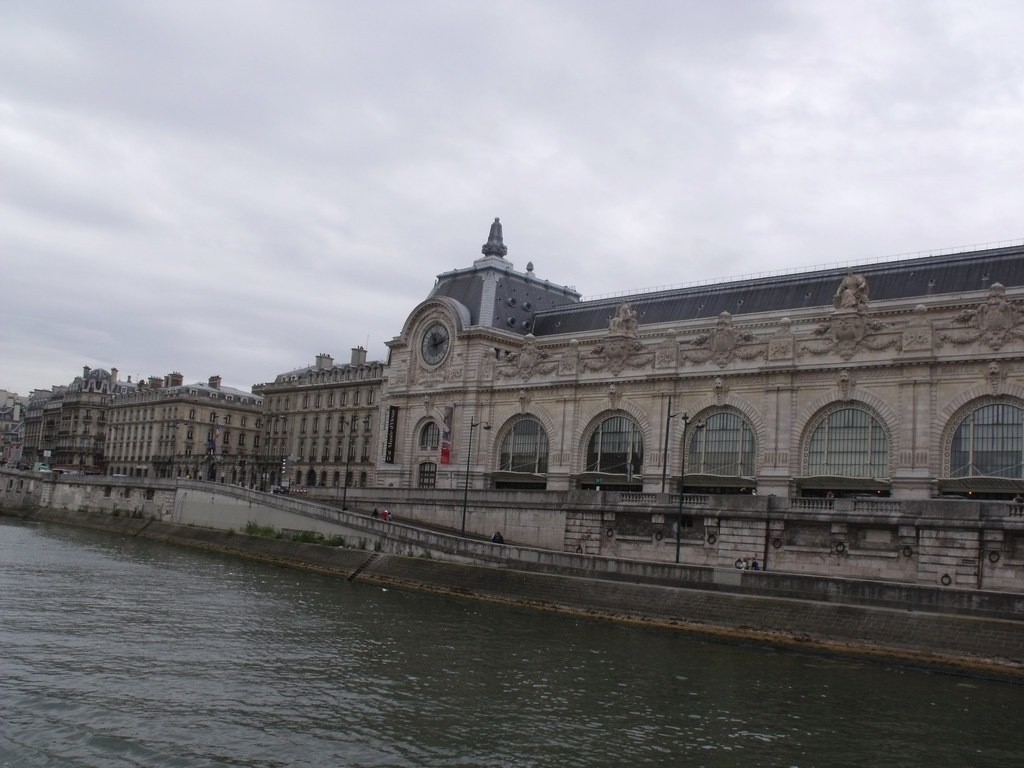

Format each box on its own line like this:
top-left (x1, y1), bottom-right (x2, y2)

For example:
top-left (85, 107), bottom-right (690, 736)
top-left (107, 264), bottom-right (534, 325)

top-left (461, 416), bottom-right (492, 538)
top-left (675, 411), bottom-right (707, 564)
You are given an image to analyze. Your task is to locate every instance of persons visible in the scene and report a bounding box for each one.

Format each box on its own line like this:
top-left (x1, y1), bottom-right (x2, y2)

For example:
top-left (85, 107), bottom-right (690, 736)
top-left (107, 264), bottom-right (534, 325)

top-left (739, 487), bottom-right (836, 510)
top-left (1013, 494), bottom-right (1024, 515)
top-left (739, 558), bottom-right (760, 570)
top-left (833, 267), bottom-right (869, 310)
top-left (372, 507), bottom-right (504, 543)
